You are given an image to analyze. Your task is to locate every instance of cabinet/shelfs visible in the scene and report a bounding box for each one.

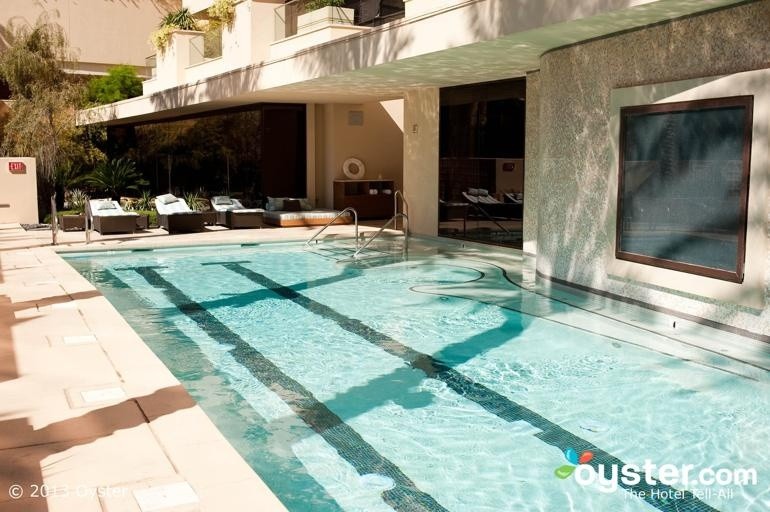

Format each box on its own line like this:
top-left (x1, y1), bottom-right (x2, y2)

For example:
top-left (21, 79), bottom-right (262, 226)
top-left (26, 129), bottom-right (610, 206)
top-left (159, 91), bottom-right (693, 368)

top-left (337, 177), bottom-right (398, 222)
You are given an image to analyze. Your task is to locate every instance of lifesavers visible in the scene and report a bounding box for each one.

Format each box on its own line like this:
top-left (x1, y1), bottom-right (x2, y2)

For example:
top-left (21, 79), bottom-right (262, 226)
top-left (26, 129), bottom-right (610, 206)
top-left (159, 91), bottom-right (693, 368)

top-left (343, 158), bottom-right (365, 180)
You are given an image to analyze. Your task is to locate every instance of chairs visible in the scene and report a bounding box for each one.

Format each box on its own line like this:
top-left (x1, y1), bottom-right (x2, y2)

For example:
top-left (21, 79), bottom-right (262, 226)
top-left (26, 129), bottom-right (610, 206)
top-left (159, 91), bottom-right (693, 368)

top-left (437, 186), bottom-right (526, 233)
top-left (64, 194), bottom-right (351, 233)
